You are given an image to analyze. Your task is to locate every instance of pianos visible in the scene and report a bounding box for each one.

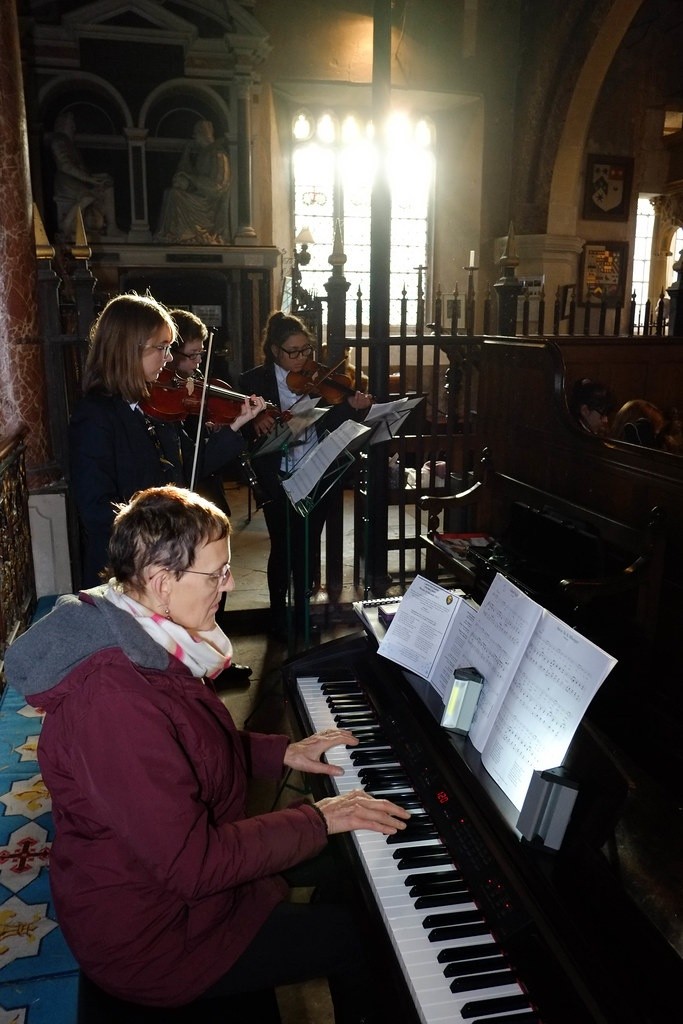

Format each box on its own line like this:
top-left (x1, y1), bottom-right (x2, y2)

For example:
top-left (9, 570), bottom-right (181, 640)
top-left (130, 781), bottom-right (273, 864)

top-left (280, 630), bottom-right (683, 1024)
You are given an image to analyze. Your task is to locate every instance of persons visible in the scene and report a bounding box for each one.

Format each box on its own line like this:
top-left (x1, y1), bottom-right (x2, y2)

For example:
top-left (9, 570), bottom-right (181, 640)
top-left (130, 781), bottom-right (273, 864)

top-left (570, 376), bottom-right (683, 456)
top-left (153, 120), bottom-right (232, 246)
top-left (47, 112), bottom-right (128, 237)
top-left (0, 484), bottom-right (422, 1024)
top-left (147, 308), bottom-right (253, 685)
top-left (68, 294), bottom-right (267, 591)
top-left (233, 312), bottom-right (373, 668)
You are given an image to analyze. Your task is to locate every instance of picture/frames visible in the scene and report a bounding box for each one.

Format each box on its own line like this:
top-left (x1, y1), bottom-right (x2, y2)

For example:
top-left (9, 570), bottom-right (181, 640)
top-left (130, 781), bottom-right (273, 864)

top-left (575, 240), bottom-right (629, 310)
top-left (580, 149), bottom-right (634, 222)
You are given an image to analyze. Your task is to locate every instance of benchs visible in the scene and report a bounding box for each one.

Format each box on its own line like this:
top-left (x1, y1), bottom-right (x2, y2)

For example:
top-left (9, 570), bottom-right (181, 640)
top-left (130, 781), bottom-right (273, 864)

top-left (78, 948), bottom-right (281, 1024)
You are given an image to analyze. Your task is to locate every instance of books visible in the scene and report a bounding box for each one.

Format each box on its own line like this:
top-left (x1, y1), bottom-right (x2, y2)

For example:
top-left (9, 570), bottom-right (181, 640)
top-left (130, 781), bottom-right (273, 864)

top-left (442, 573), bottom-right (617, 814)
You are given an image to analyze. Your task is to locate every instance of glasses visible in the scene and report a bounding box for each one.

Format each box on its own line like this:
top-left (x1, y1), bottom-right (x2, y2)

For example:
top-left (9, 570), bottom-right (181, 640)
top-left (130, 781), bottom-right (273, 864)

top-left (140, 344), bottom-right (170, 359)
top-left (173, 348), bottom-right (206, 360)
top-left (280, 344), bottom-right (314, 359)
top-left (149, 564), bottom-right (230, 588)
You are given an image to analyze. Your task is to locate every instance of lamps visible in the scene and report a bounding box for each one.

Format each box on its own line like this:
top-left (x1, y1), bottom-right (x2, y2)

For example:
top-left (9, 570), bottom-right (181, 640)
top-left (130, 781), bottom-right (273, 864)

top-left (296, 227), bottom-right (314, 266)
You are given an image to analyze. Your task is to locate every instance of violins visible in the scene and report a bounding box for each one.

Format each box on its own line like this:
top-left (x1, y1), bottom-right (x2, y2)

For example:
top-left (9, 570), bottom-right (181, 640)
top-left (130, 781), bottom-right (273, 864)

top-left (286, 359), bottom-right (378, 406)
top-left (138, 368), bottom-right (279, 426)
top-left (208, 378), bottom-right (293, 422)
